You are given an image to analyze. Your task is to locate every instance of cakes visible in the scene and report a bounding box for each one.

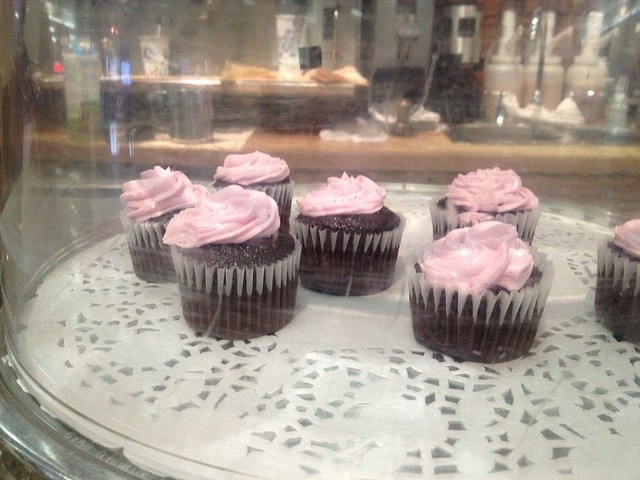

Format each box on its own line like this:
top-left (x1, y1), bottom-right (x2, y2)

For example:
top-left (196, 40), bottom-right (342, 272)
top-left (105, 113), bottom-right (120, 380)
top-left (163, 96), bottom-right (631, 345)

top-left (118, 166), bottom-right (211, 284)
top-left (408, 222), bottom-right (553, 365)
top-left (162, 184), bottom-right (303, 340)
top-left (595, 217), bottom-right (640, 343)
top-left (212, 152), bottom-right (295, 234)
top-left (295, 171), bottom-right (406, 297)
top-left (427, 168), bottom-right (544, 241)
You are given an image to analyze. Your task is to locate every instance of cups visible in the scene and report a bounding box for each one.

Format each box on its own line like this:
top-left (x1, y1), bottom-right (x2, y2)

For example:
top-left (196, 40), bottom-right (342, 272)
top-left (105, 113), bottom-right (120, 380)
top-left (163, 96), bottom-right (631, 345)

top-left (148, 87), bottom-right (214, 144)
top-left (138, 35), bottom-right (172, 77)
top-left (274, 13), bottom-right (308, 79)
